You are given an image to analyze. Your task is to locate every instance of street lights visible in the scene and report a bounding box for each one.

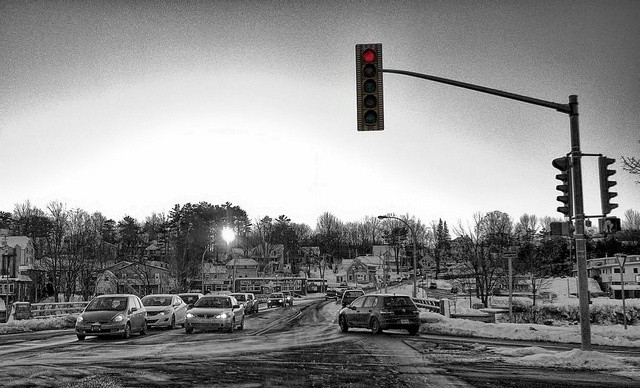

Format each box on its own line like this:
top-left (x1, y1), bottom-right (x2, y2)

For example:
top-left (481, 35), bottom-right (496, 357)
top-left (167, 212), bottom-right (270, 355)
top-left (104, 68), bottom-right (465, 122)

top-left (378, 215), bottom-right (417, 297)
top-left (201, 227), bottom-right (237, 294)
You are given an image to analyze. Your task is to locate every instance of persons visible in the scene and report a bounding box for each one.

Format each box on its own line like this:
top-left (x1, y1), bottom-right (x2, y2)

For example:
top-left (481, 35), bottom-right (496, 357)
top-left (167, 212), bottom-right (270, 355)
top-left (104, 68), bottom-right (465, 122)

top-left (116, 300), bottom-right (126, 310)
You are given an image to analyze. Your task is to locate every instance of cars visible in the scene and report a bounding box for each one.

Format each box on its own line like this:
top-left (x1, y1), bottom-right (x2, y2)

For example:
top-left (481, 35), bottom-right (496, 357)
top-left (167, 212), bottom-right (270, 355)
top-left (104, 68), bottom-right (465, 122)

top-left (230, 293), bottom-right (253, 313)
top-left (184, 295), bottom-right (245, 332)
top-left (342, 290), bottom-right (365, 306)
top-left (140, 294), bottom-right (188, 330)
top-left (246, 293), bottom-right (259, 313)
top-left (75, 294), bottom-right (147, 340)
top-left (285, 295), bottom-right (293, 306)
top-left (267, 293), bottom-right (286, 308)
top-left (178, 293), bottom-right (204, 307)
top-left (340, 294), bottom-right (419, 335)
top-left (325, 283), bottom-right (347, 301)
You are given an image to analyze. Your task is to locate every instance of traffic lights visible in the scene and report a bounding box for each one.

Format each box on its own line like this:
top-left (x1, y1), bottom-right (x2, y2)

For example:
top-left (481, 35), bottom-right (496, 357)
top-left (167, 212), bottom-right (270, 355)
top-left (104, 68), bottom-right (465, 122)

top-left (552, 157), bottom-right (573, 217)
top-left (597, 217), bottom-right (621, 234)
top-left (356, 43), bottom-right (385, 132)
top-left (599, 156), bottom-right (618, 214)
top-left (550, 222), bottom-right (569, 236)
